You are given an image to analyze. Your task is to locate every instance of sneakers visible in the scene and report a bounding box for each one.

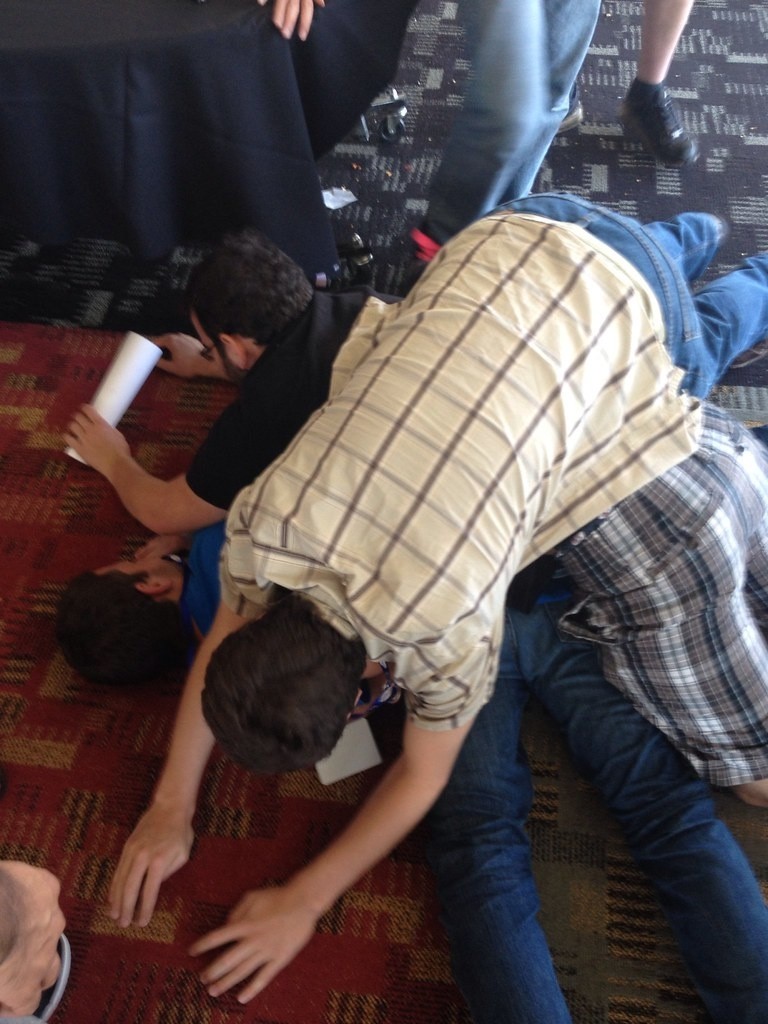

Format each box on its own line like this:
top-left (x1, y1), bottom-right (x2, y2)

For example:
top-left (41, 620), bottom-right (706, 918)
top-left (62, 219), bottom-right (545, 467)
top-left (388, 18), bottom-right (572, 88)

top-left (625, 80), bottom-right (692, 166)
top-left (405, 213), bottom-right (444, 265)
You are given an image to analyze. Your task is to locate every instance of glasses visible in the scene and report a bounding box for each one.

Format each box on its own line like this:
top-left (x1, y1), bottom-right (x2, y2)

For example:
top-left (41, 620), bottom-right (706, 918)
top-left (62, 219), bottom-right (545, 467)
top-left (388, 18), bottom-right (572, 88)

top-left (199, 331), bottom-right (220, 360)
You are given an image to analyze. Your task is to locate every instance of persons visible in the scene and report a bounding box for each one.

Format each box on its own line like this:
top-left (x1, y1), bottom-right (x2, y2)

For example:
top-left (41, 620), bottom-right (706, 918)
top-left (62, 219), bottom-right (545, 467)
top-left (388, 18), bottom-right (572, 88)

top-left (0, 860), bottom-right (71, 1024)
top-left (54, 192), bottom-right (768, 1024)
top-left (0, 0), bottom-right (701, 291)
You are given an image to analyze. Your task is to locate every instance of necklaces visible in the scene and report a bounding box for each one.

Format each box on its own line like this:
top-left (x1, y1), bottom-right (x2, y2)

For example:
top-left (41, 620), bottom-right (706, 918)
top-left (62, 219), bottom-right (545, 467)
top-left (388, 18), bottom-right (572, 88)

top-left (351, 659), bottom-right (392, 720)
